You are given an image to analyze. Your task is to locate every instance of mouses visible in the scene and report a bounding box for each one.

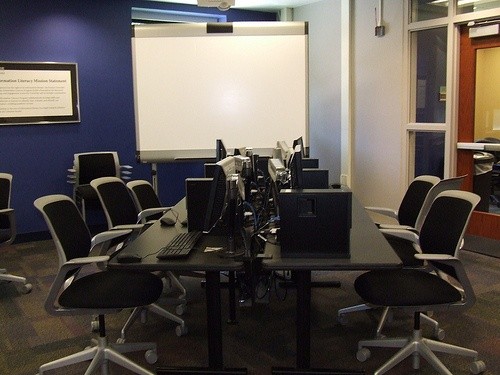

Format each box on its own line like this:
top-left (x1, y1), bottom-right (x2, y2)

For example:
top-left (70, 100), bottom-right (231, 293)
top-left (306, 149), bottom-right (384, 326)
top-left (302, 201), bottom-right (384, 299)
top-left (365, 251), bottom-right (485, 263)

top-left (117, 253), bottom-right (142, 263)
top-left (160, 217), bottom-right (176, 227)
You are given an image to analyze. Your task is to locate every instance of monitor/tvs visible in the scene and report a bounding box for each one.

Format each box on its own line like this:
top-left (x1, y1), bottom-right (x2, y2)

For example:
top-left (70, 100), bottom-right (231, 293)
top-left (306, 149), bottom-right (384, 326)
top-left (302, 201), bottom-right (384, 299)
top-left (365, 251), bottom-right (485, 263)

top-left (268, 137), bottom-right (304, 210)
top-left (202, 139), bottom-right (258, 258)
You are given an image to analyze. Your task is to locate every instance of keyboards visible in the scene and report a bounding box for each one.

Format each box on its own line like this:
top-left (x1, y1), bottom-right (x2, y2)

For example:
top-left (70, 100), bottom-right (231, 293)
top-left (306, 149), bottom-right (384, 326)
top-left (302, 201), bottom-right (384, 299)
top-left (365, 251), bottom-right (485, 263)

top-left (156, 232), bottom-right (201, 259)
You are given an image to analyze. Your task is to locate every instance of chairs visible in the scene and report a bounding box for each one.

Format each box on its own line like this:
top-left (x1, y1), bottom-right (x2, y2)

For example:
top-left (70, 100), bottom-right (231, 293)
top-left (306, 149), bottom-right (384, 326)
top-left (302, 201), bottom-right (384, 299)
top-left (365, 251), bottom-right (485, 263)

top-left (33, 195), bottom-right (159, 375)
top-left (89, 177), bottom-right (186, 343)
top-left (67, 151), bottom-right (134, 220)
top-left (126, 180), bottom-right (206, 299)
top-left (0, 173), bottom-right (33, 293)
top-left (364, 175), bottom-right (466, 301)
top-left (354, 189), bottom-right (486, 375)
top-left (476, 136), bottom-right (500, 208)
top-left (337, 173), bottom-right (470, 341)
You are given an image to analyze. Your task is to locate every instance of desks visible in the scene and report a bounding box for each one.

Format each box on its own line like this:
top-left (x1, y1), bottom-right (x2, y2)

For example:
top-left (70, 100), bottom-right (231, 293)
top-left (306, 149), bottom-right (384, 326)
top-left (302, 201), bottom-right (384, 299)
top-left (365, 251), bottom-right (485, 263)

top-left (263, 184), bottom-right (403, 375)
top-left (106, 196), bottom-right (264, 375)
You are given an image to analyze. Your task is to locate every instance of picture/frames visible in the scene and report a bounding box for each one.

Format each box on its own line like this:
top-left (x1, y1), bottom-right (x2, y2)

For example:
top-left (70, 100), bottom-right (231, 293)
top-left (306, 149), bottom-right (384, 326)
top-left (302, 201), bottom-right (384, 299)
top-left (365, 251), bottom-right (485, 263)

top-left (1, 61), bottom-right (81, 125)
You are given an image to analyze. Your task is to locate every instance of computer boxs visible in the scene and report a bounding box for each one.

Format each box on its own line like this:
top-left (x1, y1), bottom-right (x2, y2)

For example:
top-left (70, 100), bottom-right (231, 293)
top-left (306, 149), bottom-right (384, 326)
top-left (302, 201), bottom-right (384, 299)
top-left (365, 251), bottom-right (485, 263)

top-left (302, 168), bottom-right (329, 189)
top-left (302, 158), bottom-right (319, 168)
top-left (185, 178), bottom-right (214, 233)
top-left (280, 188), bottom-right (352, 259)
top-left (204, 163), bottom-right (216, 178)
